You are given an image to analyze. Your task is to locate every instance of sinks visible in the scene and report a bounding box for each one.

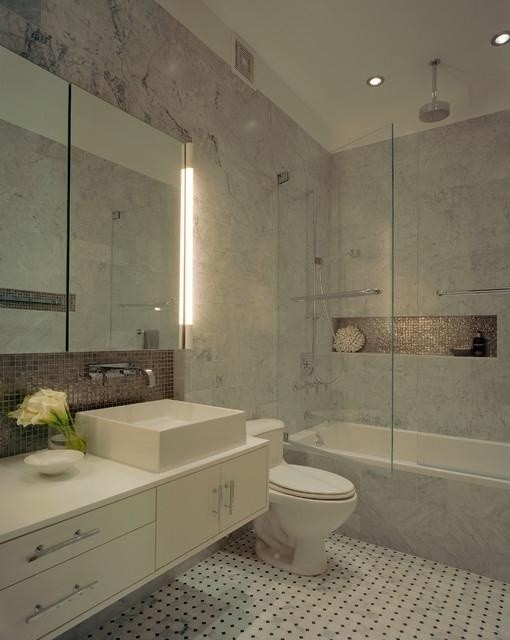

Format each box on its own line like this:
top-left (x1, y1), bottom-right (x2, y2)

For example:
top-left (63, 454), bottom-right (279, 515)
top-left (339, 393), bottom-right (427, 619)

top-left (74, 398), bottom-right (246, 475)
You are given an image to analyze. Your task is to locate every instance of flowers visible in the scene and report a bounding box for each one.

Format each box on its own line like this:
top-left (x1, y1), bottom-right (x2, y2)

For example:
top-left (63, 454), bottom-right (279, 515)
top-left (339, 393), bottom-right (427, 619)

top-left (7, 389), bottom-right (78, 429)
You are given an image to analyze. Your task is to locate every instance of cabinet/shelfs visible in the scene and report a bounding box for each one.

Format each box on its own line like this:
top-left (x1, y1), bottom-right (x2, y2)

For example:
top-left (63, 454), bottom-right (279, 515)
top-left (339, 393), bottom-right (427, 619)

top-left (69, 82), bottom-right (183, 353)
top-left (0, 484), bottom-right (157, 590)
top-left (1, 521), bottom-right (156, 640)
top-left (1, 45), bottom-right (68, 354)
top-left (221, 444), bottom-right (268, 531)
top-left (157, 463), bottom-right (221, 571)
top-left (136, 329), bottom-right (159, 335)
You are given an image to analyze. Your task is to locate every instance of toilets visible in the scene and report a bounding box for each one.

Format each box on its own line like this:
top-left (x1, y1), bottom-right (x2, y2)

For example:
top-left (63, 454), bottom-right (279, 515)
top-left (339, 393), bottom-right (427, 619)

top-left (246, 418), bottom-right (357, 576)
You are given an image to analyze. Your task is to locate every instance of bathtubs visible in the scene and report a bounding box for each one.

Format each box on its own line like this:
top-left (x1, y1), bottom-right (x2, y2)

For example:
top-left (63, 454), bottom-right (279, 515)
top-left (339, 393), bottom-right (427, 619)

top-left (282, 416), bottom-right (510, 489)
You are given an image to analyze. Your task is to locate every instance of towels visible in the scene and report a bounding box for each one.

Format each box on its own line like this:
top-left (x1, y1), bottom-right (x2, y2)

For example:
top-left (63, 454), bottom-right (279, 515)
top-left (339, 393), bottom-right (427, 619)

top-left (143, 330), bottom-right (159, 350)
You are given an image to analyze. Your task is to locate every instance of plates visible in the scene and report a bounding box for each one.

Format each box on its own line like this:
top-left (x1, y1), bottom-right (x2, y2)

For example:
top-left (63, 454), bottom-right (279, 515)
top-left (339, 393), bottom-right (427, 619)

top-left (23, 449), bottom-right (84, 476)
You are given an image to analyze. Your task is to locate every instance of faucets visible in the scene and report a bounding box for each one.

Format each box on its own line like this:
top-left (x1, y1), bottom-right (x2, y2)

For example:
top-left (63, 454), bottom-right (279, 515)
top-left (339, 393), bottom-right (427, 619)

top-left (122, 362), bottom-right (156, 388)
top-left (302, 408), bottom-right (331, 428)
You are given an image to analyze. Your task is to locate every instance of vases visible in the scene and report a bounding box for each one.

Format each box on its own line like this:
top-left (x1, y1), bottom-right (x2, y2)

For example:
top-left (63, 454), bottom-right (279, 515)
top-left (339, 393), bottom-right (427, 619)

top-left (48, 425), bottom-right (86, 452)
